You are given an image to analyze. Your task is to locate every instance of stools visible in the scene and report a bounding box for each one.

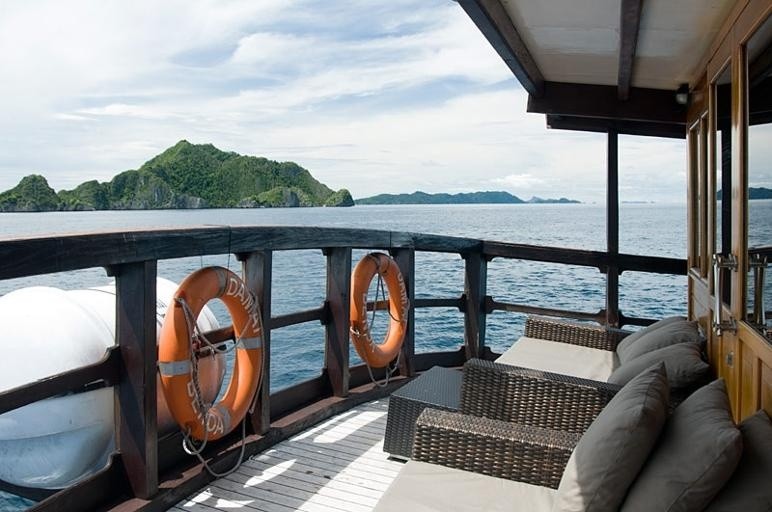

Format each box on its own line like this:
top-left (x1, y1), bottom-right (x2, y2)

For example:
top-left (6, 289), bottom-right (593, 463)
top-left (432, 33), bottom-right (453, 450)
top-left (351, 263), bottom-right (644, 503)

top-left (381, 363), bottom-right (463, 464)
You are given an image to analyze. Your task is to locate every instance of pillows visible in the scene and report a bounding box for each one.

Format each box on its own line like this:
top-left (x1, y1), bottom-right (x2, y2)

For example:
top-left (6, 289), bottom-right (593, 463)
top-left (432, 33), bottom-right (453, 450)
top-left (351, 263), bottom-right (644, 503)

top-left (556, 360), bottom-right (772, 512)
top-left (607, 316), bottom-right (710, 385)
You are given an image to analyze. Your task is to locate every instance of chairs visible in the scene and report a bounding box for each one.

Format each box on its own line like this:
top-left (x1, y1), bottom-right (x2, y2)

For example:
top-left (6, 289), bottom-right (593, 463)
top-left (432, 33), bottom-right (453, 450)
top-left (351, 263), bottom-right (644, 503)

top-left (369, 407), bottom-right (583, 512)
top-left (463, 315), bottom-right (634, 457)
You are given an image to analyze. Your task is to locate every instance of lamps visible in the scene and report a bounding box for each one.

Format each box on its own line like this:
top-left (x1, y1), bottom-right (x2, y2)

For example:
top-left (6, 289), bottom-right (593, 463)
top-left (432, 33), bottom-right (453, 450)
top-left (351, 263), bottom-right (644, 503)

top-left (674, 82), bottom-right (691, 104)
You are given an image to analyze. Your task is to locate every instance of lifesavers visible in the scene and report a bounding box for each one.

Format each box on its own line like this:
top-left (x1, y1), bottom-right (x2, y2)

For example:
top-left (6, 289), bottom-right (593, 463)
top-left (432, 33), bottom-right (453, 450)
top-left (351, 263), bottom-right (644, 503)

top-left (158, 266), bottom-right (264, 441)
top-left (350, 251), bottom-right (409, 367)
